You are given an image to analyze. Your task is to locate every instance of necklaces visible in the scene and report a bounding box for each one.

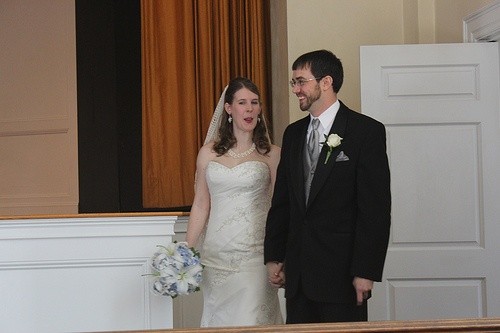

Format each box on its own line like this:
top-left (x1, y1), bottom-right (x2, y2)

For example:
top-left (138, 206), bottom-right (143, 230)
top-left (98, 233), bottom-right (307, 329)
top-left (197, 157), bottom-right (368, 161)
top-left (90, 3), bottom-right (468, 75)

top-left (225, 142), bottom-right (256, 159)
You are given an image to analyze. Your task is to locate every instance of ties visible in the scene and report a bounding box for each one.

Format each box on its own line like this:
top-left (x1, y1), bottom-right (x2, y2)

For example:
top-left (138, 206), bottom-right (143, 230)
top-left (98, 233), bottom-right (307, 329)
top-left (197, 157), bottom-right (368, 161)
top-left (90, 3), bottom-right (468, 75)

top-left (304, 117), bottom-right (321, 205)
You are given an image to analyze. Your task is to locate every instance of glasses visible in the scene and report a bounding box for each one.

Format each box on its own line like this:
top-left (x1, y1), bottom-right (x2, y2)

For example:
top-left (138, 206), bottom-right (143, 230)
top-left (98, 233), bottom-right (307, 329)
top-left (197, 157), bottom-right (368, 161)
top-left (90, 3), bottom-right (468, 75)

top-left (290, 78), bottom-right (321, 87)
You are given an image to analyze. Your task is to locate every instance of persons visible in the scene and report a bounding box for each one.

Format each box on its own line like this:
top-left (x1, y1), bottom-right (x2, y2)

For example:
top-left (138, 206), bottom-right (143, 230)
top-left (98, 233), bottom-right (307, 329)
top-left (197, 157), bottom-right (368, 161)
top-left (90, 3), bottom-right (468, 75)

top-left (186, 77), bottom-right (286, 329)
top-left (263, 49), bottom-right (391, 324)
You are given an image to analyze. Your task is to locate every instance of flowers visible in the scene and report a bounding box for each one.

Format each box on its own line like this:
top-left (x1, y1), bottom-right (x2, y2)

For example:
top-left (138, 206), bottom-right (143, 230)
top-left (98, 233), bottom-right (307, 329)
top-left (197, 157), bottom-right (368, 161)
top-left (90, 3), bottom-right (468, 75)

top-left (142, 240), bottom-right (210, 303)
top-left (319, 133), bottom-right (343, 165)
